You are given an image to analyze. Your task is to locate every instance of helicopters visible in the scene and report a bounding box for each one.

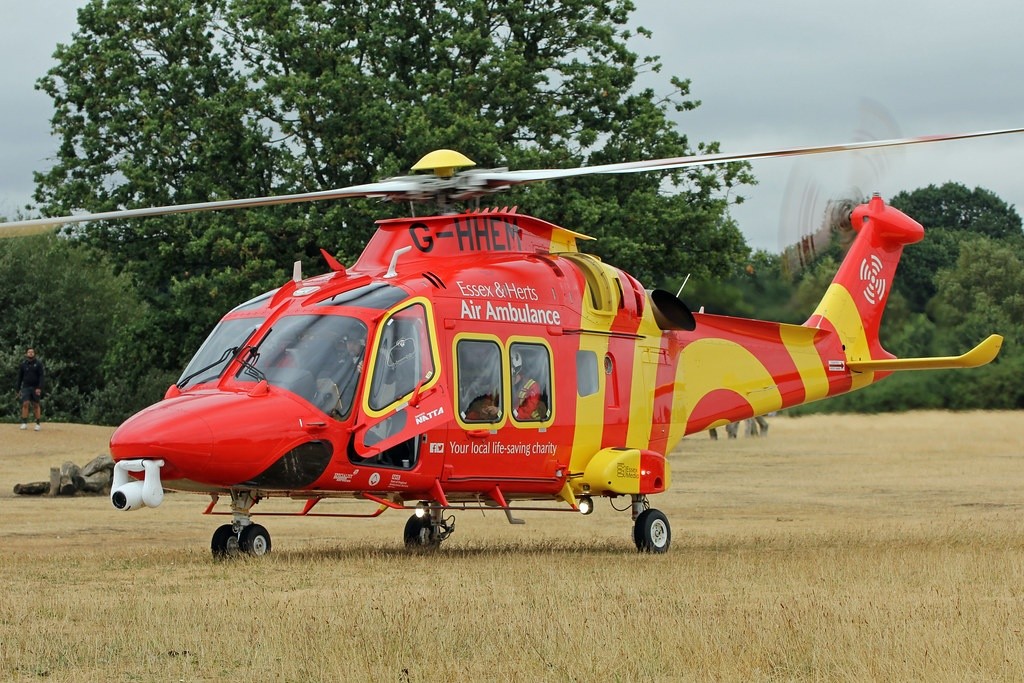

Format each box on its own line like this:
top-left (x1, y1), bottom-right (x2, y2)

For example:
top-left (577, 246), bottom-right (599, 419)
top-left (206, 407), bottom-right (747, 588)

top-left (0, 128), bottom-right (1024, 559)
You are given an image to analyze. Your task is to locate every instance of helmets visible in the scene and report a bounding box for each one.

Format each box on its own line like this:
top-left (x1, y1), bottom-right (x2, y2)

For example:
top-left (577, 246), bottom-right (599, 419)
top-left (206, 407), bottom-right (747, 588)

top-left (342, 323), bottom-right (368, 341)
top-left (509, 350), bottom-right (523, 376)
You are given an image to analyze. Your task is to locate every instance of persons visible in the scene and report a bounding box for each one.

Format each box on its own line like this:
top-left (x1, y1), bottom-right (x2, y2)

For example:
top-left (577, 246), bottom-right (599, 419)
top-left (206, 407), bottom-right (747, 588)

top-left (329, 333), bottom-right (396, 411)
top-left (15, 347), bottom-right (44, 432)
top-left (466, 351), bottom-right (540, 421)
